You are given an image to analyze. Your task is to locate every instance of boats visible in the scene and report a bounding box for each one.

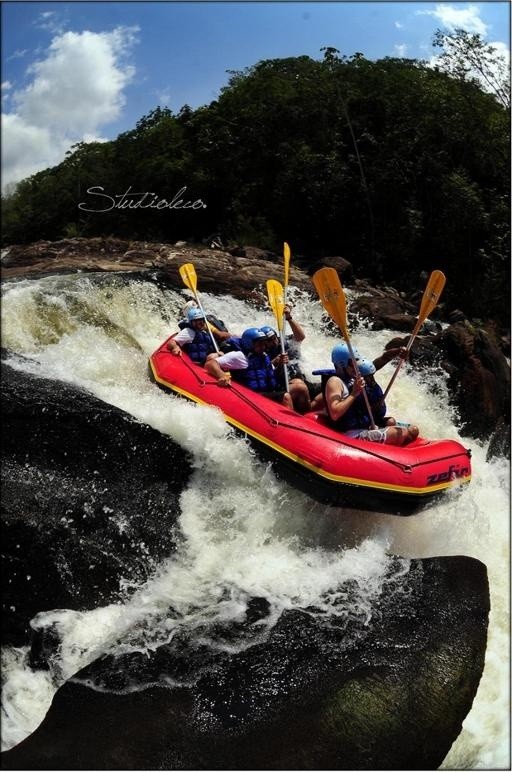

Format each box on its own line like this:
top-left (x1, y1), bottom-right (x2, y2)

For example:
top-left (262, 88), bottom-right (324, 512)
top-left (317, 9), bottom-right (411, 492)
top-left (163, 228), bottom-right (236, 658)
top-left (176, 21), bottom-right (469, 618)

top-left (145, 330), bottom-right (477, 518)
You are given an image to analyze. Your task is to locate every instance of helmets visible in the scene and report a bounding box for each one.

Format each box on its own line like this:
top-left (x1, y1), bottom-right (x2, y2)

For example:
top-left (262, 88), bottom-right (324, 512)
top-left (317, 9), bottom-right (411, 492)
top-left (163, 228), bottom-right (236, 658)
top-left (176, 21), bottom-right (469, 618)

top-left (242, 326), bottom-right (277, 352)
top-left (182, 301), bottom-right (204, 321)
top-left (332, 344), bottom-right (376, 376)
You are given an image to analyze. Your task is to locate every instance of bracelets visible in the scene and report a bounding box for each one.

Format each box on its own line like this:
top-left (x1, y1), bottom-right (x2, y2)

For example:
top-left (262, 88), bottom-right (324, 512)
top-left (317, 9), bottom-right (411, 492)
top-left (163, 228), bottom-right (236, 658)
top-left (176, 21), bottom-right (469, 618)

top-left (286, 317), bottom-right (292, 320)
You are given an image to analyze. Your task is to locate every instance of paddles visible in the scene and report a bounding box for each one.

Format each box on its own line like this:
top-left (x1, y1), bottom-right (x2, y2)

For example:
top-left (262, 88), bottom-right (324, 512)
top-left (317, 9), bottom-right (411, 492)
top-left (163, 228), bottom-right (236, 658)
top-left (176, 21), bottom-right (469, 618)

top-left (266, 242), bottom-right (290, 392)
top-left (384, 270), bottom-right (446, 401)
top-left (179, 263), bottom-right (220, 353)
top-left (313, 268), bottom-right (375, 431)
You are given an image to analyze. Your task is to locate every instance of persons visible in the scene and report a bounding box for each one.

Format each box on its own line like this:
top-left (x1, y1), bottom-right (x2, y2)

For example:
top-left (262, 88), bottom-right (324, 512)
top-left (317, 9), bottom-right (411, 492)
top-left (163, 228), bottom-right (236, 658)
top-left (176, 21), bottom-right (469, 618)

top-left (183, 300), bottom-right (231, 340)
top-left (357, 347), bottom-right (419, 445)
top-left (259, 306), bottom-right (323, 411)
top-left (205, 327), bottom-right (311, 418)
top-left (325, 343), bottom-right (409, 447)
top-left (167, 308), bottom-right (224, 366)
